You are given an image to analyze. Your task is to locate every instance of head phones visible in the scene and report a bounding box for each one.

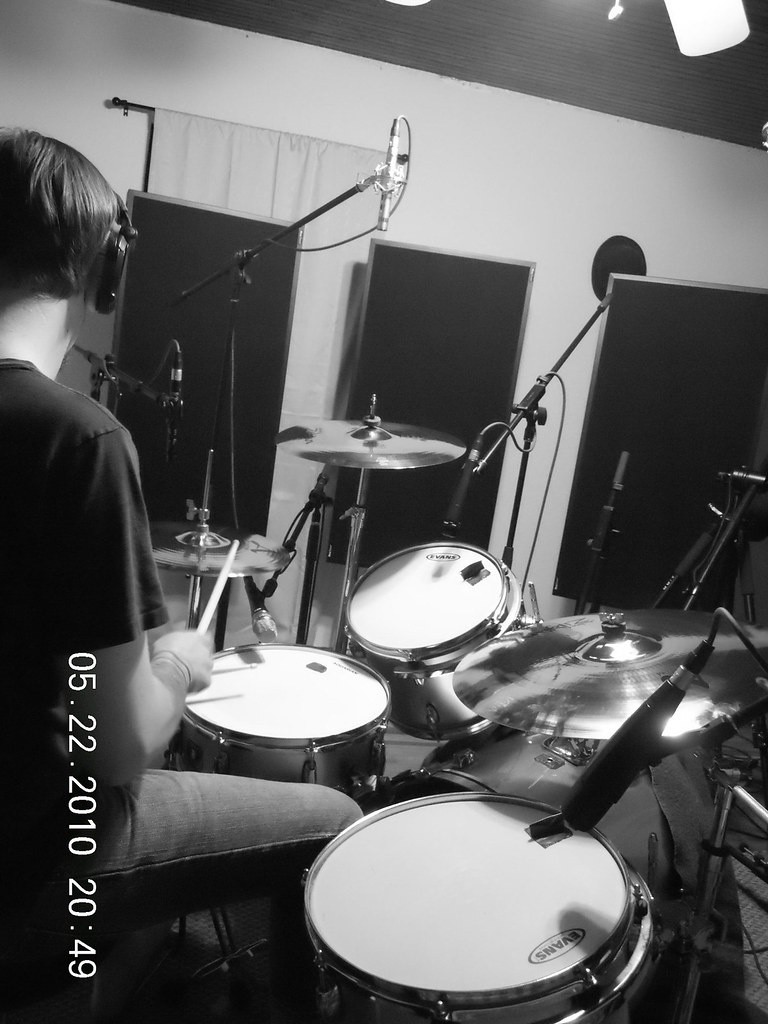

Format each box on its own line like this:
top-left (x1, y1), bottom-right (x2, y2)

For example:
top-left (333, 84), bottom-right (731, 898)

top-left (83, 191), bottom-right (137, 316)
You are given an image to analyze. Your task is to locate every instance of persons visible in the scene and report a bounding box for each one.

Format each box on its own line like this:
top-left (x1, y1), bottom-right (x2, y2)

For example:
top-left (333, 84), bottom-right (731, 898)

top-left (0, 130), bottom-right (363, 1024)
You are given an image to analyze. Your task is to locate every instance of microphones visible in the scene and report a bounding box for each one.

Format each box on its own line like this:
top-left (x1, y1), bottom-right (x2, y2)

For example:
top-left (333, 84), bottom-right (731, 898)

top-left (241, 574), bottom-right (278, 644)
top-left (732, 470), bottom-right (768, 485)
top-left (375, 118), bottom-right (400, 231)
top-left (165, 353), bottom-right (185, 466)
top-left (559, 641), bottom-right (716, 832)
top-left (441, 434), bottom-right (485, 538)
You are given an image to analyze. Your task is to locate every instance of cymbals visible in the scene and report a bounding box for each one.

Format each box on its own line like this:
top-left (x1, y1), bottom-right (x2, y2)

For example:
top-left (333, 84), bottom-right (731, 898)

top-left (451, 608), bottom-right (767, 745)
top-left (145, 521), bottom-right (291, 580)
top-left (282, 418), bottom-right (465, 472)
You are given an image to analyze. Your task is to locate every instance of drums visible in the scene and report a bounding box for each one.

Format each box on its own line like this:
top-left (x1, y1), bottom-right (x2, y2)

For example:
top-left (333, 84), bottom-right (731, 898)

top-left (338, 541), bottom-right (519, 738)
top-left (357, 722), bottom-right (706, 939)
top-left (301, 787), bottom-right (667, 1024)
top-left (169, 642), bottom-right (394, 786)
